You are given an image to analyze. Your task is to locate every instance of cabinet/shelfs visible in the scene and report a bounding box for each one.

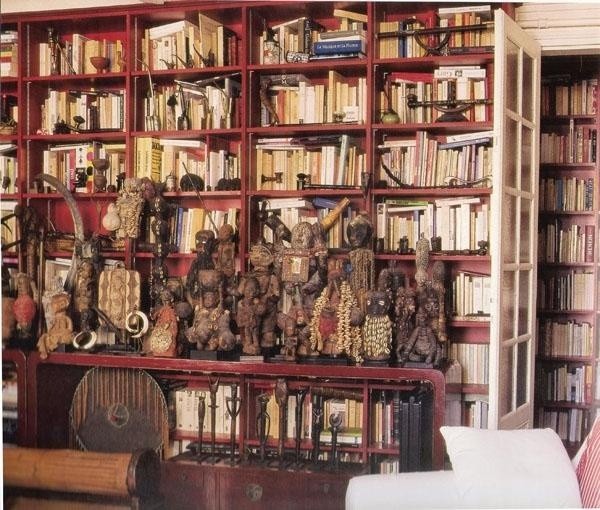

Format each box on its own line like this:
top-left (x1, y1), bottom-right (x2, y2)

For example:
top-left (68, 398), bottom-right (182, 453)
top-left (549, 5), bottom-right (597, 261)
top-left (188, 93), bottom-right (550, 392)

top-left (2, 3), bottom-right (600, 508)
